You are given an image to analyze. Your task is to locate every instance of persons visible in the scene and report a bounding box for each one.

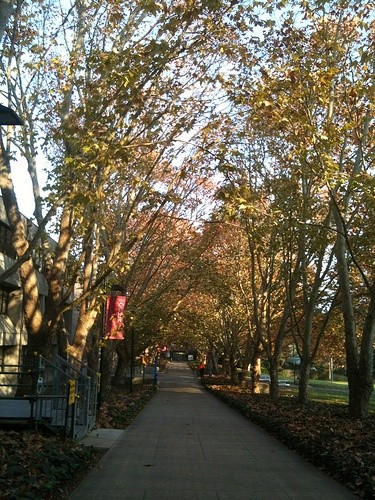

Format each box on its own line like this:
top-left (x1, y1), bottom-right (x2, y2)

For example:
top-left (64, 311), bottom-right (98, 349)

top-left (188, 355), bottom-right (205, 379)
